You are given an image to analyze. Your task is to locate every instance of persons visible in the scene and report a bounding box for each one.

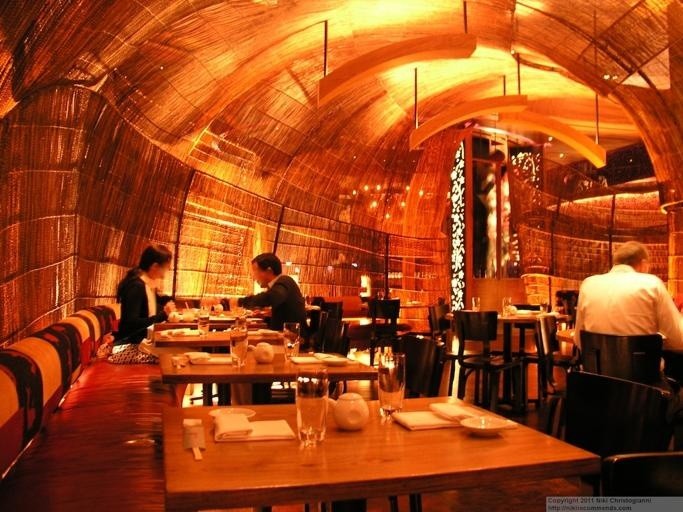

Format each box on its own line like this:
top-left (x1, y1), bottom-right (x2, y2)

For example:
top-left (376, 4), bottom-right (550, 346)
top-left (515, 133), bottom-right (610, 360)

top-left (237, 251), bottom-right (309, 404)
top-left (573, 240), bottom-right (683, 391)
top-left (108, 245), bottom-right (189, 408)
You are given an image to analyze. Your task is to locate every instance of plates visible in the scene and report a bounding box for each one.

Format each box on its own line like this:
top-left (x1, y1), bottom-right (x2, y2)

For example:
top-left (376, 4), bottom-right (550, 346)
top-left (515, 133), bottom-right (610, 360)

top-left (460, 418), bottom-right (513, 436)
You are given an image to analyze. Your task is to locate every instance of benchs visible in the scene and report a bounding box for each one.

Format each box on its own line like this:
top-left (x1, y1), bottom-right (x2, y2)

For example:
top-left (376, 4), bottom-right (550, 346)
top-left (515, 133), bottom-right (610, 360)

top-left (170, 294), bottom-right (414, 350)
top-left (0, 304), bottom-right (178, 512)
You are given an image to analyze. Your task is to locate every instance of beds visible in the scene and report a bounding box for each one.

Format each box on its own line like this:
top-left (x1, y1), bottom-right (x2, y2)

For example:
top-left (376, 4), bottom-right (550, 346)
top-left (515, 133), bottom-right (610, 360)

top-left (161, 395), bottom-right (602, 511)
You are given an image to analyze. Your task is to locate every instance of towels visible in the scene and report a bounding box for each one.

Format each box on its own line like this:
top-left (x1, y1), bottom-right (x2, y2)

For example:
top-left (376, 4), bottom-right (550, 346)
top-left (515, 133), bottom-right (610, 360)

top-left (289, 352), bottom-right (337, 365)
top-left (390, 402), bottom-right (478, 432)
top-left (188, 352), bottom-right (231, 367)
top-left (210, 412), bottom-right (296, 444)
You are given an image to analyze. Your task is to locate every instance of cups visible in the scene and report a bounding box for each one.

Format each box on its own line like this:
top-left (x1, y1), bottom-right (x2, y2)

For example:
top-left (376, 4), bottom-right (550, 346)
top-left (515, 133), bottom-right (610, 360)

top-left (198, 311), bottom-right (406, 447)
top-left (472, 297), bottom-right (547, 317)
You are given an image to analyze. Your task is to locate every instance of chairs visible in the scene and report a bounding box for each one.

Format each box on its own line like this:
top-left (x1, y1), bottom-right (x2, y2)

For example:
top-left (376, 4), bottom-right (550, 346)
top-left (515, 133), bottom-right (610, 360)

top-left (428, 302), bottom-right (450, 332)
top-left (318, 300), bottom-right (344, 319)
top-left (365, 297), bottom-right (402, 366)
top-left (450, 309), bottom-right (523, 417)
top-left (535, 313), bottom-right (575, 401)
top-left (400, 334), bottom-right (447, 401)
top-left (565, 366), bottom-right (672, 458)
top-left (577, 328), bottom-right (667, 386)
top-left (314, 311), bottom-right (350, 359)
